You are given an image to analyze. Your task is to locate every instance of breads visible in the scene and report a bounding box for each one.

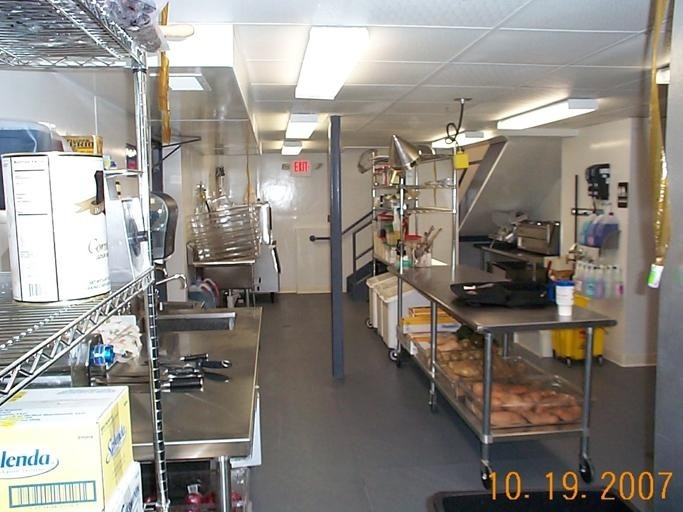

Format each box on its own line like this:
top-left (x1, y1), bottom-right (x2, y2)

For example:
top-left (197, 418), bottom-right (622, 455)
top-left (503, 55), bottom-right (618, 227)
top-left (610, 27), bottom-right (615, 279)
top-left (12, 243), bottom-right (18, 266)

top-left (418, 332), bottom-right (581, 428)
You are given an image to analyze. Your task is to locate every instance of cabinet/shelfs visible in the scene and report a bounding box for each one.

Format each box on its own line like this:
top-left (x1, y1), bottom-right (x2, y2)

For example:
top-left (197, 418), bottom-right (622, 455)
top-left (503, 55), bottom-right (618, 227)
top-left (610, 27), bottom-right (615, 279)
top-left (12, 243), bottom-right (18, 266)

top-left (368, 143), bottom-right (618, 489)
top-left (0, 0), bottom-right (263, 512)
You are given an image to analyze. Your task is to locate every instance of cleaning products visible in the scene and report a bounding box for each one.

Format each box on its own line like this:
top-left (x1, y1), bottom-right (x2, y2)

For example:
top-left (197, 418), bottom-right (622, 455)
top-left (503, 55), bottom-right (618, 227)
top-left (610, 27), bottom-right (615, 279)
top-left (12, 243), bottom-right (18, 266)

top-left (571, 260), bottom-right (624, 299)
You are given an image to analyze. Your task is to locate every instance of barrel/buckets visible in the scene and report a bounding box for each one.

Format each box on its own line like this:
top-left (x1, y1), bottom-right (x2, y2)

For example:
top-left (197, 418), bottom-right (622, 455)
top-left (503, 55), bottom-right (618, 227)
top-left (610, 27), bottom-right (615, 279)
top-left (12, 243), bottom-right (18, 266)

top-left (580, 211), bottom-right (597, 244)
top-left (189, 275), bottom-right (221, 309)
top-left (587, 210), bottom-right (618, 249)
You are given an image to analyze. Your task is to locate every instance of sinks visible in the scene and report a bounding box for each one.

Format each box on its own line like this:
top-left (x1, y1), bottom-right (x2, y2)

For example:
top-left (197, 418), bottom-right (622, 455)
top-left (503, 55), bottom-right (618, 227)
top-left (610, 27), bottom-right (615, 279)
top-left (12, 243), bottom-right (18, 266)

top-left (137, 312), bottom-right (237, 331)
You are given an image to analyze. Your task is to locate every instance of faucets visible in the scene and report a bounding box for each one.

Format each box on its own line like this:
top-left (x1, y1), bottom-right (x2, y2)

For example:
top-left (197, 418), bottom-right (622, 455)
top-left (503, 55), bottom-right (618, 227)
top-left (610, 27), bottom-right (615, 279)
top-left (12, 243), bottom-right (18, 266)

top-left (154, 267), bottom-right (188, 289)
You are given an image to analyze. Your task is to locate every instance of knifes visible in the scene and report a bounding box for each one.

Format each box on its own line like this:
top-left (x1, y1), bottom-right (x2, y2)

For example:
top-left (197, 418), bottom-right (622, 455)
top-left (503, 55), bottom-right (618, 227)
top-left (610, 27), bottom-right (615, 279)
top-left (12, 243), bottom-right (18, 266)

top-left (105, 353), bottom-right (230, 389)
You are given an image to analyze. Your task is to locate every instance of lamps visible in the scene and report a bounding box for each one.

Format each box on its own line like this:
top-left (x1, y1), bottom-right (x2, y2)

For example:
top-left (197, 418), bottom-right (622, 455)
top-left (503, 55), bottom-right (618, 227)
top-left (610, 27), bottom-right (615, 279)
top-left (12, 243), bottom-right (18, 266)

top-left (431, 98), bottom-right (601, 149)
top-left (294, 25), bottom-right (374, 100)
top-left (278, 112), bottom-right (322, 156)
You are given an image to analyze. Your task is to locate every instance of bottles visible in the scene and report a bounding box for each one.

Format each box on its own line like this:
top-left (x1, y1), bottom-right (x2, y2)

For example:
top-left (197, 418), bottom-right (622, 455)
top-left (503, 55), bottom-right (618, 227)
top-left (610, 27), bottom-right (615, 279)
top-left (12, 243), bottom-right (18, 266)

top-left (395, 240), bottom-right (410, 274)
top-left (648, 257), bottom-right (664, 288)
top-left (572, 258), bottom-right (624, 297)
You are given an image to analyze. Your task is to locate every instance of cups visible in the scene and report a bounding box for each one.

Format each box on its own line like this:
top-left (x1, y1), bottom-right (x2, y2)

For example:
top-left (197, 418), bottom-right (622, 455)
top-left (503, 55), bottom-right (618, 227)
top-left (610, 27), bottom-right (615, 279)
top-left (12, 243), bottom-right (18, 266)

top-left (555, 281), bottom-right (575, 317)
